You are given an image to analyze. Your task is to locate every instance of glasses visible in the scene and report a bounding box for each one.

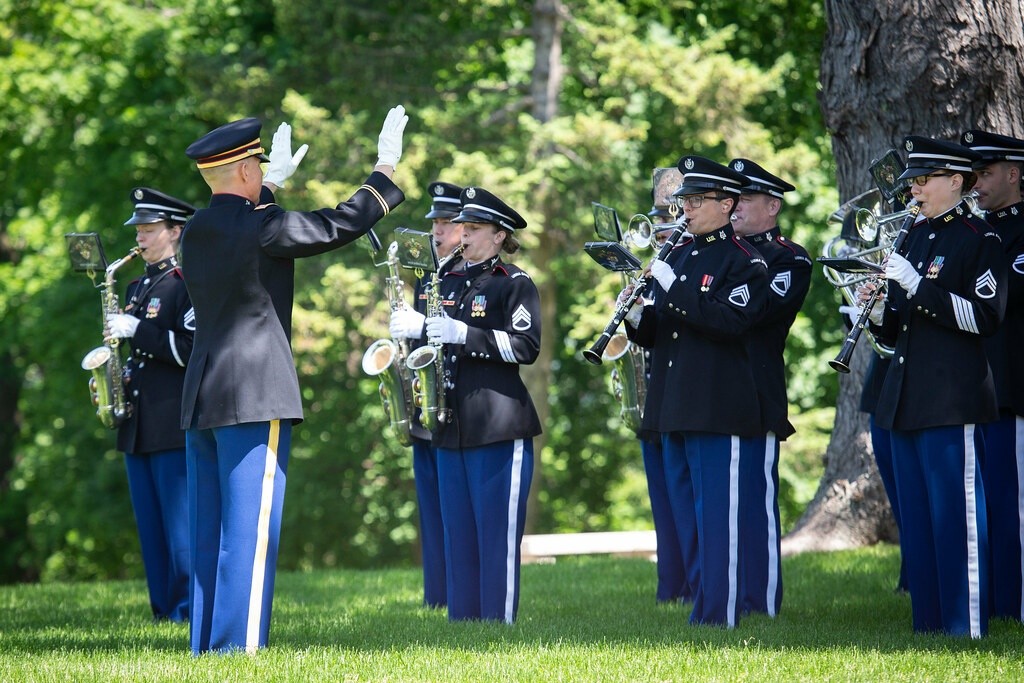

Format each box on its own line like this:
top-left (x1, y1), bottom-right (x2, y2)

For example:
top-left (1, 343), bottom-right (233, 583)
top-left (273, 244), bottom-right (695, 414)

top-left (676, 196), bottom-right (727, 208)
top-left (907, 173), bottom-right (955, 186)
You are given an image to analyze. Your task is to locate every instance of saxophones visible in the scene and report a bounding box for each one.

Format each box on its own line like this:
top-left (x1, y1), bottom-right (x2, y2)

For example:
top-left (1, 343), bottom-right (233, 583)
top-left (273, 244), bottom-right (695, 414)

top-left (601, 231), bottom-right (663, 434)
top-left (404, 243), bottom-right (470, 434)
top-left (80, 244), bottom-right (147, 430)
top-left (359, 239), bottom-right (441, 449)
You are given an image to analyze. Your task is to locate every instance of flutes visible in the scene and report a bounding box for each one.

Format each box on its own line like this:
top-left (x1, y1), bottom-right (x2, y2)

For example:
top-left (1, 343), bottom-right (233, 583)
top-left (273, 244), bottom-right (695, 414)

top-left (826, 200), bottom-right (923, 374)
top-left (581, 217), bottom-right (691, 367)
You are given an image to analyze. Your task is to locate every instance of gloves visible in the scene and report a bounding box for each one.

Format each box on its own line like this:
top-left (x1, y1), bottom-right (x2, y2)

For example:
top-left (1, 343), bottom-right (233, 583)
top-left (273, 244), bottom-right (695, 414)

top-left (425, 309), bottom-right (467, 346)
top-left (389, 302), bottom-right (425, 340)
top-left (263, 122), bottom-right (310, 190)
top-left (374, 104), bottom-right (410, 173)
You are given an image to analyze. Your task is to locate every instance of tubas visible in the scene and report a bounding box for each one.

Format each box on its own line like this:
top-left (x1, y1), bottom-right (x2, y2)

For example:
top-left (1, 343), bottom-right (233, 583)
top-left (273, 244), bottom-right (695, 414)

top-left (818, 186), bottom-right (899, 359)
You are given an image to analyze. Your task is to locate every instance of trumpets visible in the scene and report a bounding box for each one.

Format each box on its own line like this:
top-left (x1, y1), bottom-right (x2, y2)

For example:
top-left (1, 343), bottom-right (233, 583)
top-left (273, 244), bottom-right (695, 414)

top-left (627, 213), bottom-right (738, 250)
top-left (855, 190), bottom-right (983, 243)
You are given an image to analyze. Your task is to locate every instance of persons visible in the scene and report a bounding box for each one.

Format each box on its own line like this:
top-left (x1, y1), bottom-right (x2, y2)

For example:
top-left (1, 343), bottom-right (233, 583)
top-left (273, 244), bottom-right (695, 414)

top-left (181, 104), bottom-right (409, 653)
top-left (389, 182), bottom-right (541, 620)
top-left (856, 130), bottom-right (1024, 638)
top-left (616, 155), bottom-right (813, 631)
top-left (103, 188), bottom-right (198, 623)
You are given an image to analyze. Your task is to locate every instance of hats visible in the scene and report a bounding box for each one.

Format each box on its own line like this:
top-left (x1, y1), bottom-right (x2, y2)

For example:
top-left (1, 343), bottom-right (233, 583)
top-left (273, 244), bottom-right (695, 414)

top-left (897, 135), bottom-right (981, 181)
top-left (671, 156), bottom-right (752, 197)
top-left (185, 118), bottom-right (271, 169)
top-left (423, 181), bottom-right (464, 219)
top-left (727, 158), bottom-right (796, 200)
top-left (959, 128), bottom-right (1024, 171)
top-left (450, 187), bottom-right (528, 234)
top-left (124, 187), bottom-right (197, 226)
